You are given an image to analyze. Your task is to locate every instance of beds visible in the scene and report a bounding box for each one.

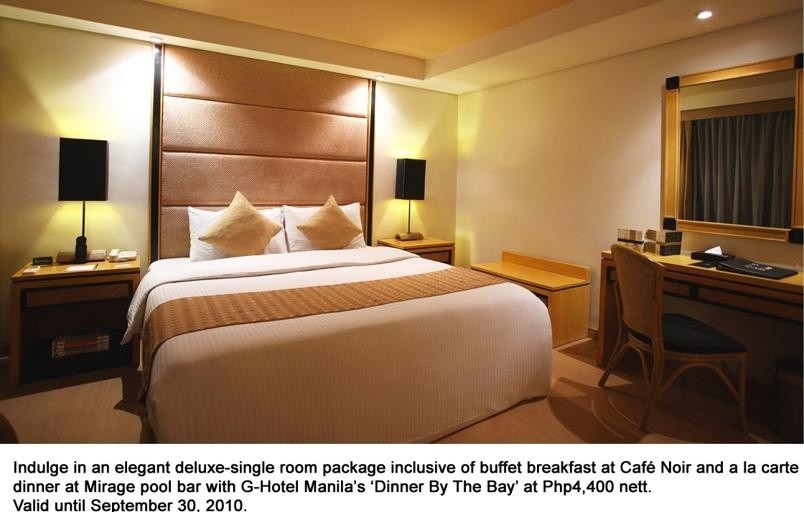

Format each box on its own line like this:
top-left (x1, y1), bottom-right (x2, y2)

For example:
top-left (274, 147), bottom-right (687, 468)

top-left (121, 43), bottom-right (551, 443)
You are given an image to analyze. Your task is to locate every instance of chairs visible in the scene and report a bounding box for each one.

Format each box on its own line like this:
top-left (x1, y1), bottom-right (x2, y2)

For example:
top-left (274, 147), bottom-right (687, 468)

top-left (598, 244), bottom-right (750, 434)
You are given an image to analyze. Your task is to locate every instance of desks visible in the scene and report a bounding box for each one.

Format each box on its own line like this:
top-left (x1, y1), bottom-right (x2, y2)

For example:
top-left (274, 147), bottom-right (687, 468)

top-left (471, 250), bottom-right (592, 348)
top-left (598, 250), bottom-right (803, 370)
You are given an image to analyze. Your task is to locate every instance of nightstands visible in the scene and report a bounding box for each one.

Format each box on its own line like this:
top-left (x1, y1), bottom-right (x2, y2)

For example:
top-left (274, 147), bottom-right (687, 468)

top-left (9, 257), bottom-right (145, 387)
top-left (378, 237), bottom-right (455, 266)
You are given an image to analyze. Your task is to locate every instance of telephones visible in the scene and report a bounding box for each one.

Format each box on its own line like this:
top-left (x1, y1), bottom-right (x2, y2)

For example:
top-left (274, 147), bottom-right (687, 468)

top-left (109, 248), bottom-right (135, 261)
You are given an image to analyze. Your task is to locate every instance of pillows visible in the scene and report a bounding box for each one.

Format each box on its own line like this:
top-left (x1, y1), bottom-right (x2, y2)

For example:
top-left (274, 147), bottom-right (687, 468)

top-left (187, 206), bottom-right (287, 263)
top-left (283, 202), bottom-right (365, 252)
top-left (297, 195), bottom-right (361, 250)
top-left (198, 190), bottom-right (282, 257)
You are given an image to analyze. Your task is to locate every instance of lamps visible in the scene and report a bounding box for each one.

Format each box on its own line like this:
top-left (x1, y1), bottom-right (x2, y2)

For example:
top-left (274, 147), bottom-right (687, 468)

top-left (58, 137), bottom-right (109, 264)
top-left (394, 159), bottom-right (426, 240)
